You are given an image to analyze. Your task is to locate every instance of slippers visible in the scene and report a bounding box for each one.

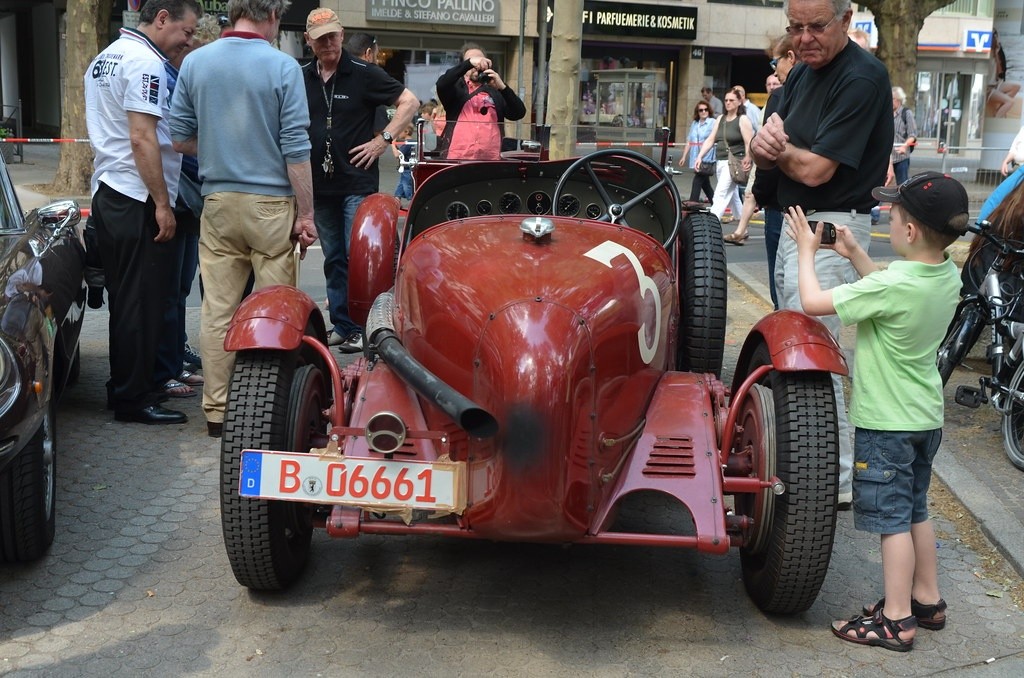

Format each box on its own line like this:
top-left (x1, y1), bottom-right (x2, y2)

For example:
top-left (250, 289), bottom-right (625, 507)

top-left (166, 380), bottom-right (197, 397)
top-left (178, 371), bottom-right (205, 385)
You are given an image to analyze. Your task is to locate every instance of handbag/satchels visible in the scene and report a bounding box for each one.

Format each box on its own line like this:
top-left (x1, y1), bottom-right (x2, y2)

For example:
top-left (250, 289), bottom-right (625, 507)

top-left (727, 150), bottom-right (752, 184)
top-left (698, 162), bottom-right (714, 175)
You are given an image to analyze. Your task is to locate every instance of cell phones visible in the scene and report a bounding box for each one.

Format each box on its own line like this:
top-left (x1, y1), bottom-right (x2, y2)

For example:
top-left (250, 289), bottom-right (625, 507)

top-left (807, 221), bottom-right (836, 244)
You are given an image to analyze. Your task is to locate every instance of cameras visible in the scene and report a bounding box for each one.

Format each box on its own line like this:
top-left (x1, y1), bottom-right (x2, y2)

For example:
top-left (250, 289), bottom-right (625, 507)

top-left (478, 70), bottom-right (491, 85)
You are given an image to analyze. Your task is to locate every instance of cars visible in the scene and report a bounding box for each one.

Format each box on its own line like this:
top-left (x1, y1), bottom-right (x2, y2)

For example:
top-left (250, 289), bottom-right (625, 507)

top-left (0, 147), bottom-right (90, 563)
top-left (219, 118), bottom-right (849, 617)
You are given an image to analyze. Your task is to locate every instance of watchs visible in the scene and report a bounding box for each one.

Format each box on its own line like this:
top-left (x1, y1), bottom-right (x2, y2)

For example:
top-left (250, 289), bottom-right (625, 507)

top-left (379, 131), bottom-right (393, 144)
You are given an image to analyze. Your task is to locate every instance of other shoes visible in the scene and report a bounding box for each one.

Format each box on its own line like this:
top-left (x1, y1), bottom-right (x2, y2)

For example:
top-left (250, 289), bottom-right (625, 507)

top-left (184, 344), bottom-right (202, 370)
top-left (207, 421), bottom-right (223, 437)
top-left (327, 327), bottom-right (363, 353)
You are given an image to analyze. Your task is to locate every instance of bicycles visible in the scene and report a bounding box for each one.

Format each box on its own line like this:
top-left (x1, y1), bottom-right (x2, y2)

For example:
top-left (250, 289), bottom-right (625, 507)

top-left (936, 220), bottom-right (1023, 471)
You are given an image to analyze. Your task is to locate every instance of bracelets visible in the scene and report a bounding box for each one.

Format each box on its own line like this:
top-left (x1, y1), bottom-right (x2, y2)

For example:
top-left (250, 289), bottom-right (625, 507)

top-left (750, 135), bottom-right (756, 154)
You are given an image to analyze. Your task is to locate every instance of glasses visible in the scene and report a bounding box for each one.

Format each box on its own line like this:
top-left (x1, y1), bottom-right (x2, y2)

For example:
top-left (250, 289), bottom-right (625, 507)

top-left (697, 108), bottom-right (708, 113)
top-left (725, 99), bottom-right (738, 103)
top-left (785, 14), bottom-right (837, 34)
top-left (366, 35), bottom-right (378, 54)
top-left (770, 52), bottom-right (788, 71)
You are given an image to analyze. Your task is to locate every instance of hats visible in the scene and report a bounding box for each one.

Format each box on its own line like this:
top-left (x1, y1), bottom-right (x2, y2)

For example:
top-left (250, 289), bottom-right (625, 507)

top-left (871, 172), bottom-right (968, 237)
top-left (307, 8), bottom-right (343, 40)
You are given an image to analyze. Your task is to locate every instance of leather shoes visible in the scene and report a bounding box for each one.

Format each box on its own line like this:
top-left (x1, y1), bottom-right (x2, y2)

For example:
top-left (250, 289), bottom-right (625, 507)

top-left (107, 388), bottom-right (169, 407)
top-left (114, 403), bottom-right (187, 423)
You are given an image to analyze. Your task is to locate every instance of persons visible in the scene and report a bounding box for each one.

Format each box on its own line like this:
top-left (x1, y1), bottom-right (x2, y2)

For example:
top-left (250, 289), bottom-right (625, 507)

top-left (989, 28), bottom-right (1021, 118)
top-left (82, 0), bottom-right (527, 437)
top-left (0, 228), bottom-right (77, 409)
top-left (749, 0), bottom-right (894, 514)
top-left (1001, 126), bottom-right (1024, 176)
top-left (784, 171), bottom-right (970, 652)
top-left (679, 33), bottom-right (917, 312)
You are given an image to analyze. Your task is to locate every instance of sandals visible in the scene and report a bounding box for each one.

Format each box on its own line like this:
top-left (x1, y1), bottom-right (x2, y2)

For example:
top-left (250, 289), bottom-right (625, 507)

top-left (723, 234), bottom-right (746, 245)
top-left (832, 611), bottom-right (917, 652)
top-left (864, 598), bottom-right (948, 631)
